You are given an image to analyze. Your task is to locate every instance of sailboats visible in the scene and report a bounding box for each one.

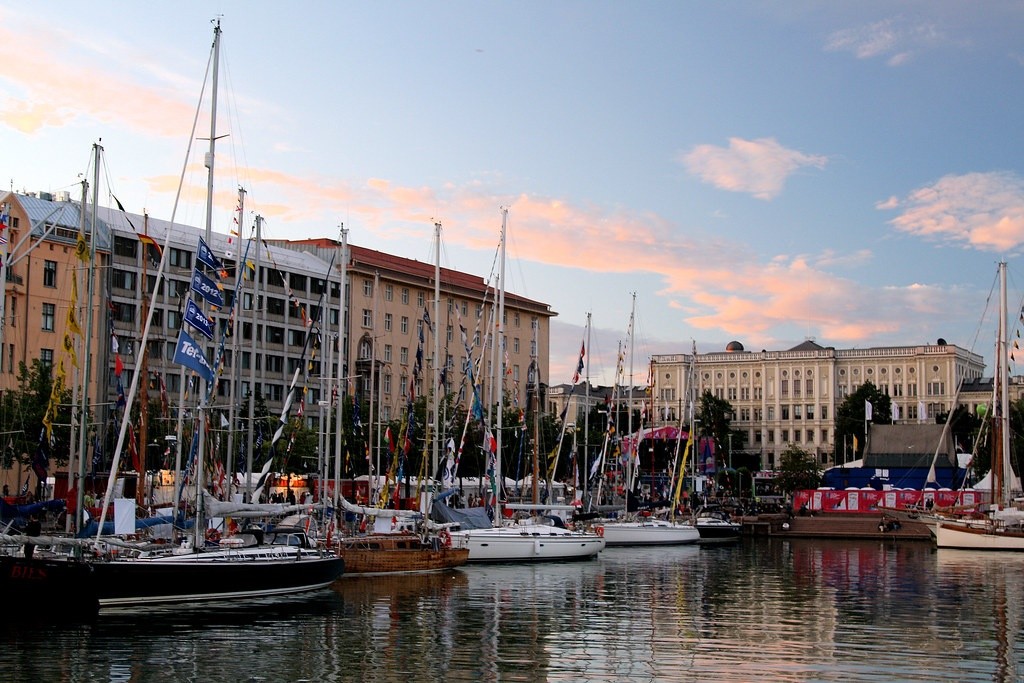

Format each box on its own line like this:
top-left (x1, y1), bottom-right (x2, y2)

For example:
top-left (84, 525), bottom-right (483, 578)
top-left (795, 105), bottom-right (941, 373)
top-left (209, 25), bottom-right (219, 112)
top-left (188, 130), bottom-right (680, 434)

top-left (0, 11), bottom-right (741, 608)
top-left (893, 255), bottom-right (1024, 550)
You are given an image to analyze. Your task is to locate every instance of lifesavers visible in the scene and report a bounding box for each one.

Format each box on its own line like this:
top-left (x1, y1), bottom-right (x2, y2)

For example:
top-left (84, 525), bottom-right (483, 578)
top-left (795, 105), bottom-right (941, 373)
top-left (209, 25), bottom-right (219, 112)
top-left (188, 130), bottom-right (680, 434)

top-left (205, 528), bottom-right (221, 542)
top-left (439, 530), bottom-right (452, 548)
top-left (596, 526), bottom-right (604, 537)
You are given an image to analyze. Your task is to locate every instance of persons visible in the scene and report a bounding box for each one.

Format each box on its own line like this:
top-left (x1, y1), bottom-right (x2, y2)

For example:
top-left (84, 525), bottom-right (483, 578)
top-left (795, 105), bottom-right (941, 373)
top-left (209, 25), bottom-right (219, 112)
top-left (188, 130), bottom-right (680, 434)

top-left (20, 512), bottom-right (41, 558)
top-left (259, 488), bottom-right (306, 505)
top-left (912, 497), bottom-right (934, 511)
top-left (878, 517), bottom-right (901, 531)
top-left (84, 491), bottom-right (105, 508)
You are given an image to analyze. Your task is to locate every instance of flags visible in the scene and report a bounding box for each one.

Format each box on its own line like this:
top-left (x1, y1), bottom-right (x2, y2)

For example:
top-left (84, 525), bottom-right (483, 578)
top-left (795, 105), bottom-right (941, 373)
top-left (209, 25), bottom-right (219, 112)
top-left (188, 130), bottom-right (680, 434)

top-left (378, 272), bottom-right (654, 510)
top-left (43, 231), bottom-right (91, 448)
top-left (107, 294), bottom-right (139, 470)
top-left (173, 206), bottom-right (313, 496)
